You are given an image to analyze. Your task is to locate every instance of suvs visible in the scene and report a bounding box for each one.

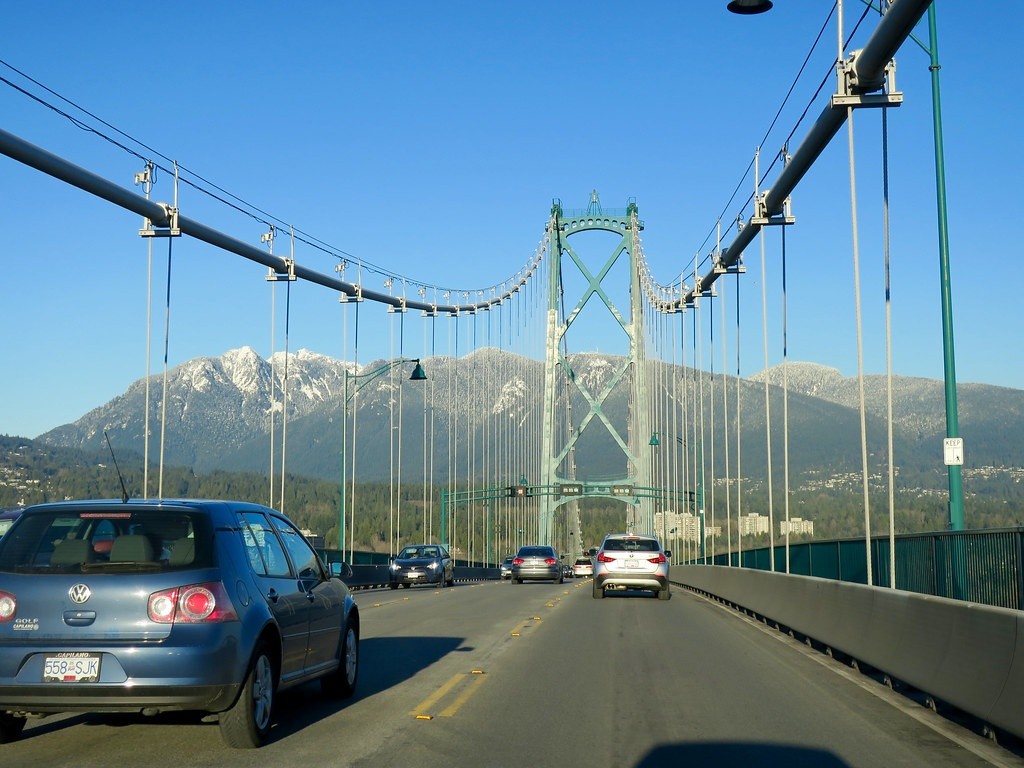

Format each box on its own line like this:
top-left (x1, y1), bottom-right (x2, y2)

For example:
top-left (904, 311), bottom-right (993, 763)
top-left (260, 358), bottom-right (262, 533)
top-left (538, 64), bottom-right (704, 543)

top-left (589, 533), bottom-right (673, 600)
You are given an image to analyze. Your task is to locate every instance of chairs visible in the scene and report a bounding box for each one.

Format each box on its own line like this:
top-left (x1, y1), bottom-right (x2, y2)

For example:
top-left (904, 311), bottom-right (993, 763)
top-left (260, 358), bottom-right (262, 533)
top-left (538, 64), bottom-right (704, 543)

top-left (613, 545), bottom-right (625, 551)
top-left (638, 546), bottom-right (651, 551)
top-left (50, 536), bottom-right (196, 567)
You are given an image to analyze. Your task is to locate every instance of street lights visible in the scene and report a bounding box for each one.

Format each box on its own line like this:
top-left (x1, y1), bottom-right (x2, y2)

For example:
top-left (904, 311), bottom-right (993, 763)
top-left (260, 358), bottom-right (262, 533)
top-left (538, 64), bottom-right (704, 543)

top-left (649, 432), bottom-right (705, 558)
top-left (487, 474), bottom-right (529, 564)
top-left (729, 0), bottom-right (967, 598)
top-left (340, 358), bottom-right (427, 550)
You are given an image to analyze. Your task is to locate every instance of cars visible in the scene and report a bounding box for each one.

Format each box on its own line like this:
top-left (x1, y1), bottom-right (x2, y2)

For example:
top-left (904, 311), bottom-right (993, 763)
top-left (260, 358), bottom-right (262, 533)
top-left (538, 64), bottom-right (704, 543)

top-left (573, 558), bottom-right (594, 578)
top-left (500, 556), bottom-right (515, 580)
top-left (0, 499), bottom-right (359, 750)
top-left (389, 545), bottom-right (455, 589)
top-left (511, 546), bottom-right (565, 584)
top-left (562, 565), bottom-right (574, 578)
